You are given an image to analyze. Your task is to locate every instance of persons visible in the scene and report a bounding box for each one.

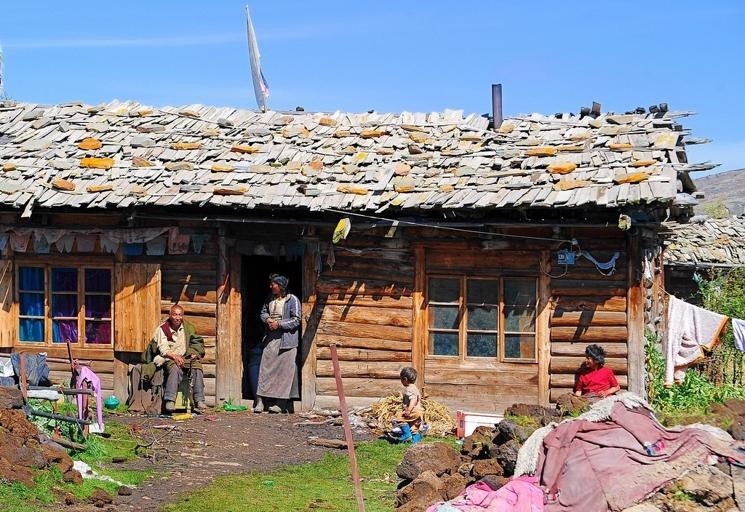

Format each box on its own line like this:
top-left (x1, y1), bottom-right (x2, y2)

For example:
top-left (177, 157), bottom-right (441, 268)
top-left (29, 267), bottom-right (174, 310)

top-left (152, 304), bottom-right (211, 412)
top-left (252, 272), bottom-right (302, 414)
top-left (397, 366), bottom-right (422, 445)
top-left (574, 344), bottom-right (621, 400)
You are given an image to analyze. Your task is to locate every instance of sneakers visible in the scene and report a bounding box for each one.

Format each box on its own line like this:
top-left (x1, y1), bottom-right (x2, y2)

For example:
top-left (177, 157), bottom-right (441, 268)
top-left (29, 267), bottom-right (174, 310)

top-left (165, 400), bottom-right (176, 411)
top-left (269, 400), bottom-right (287, 412)
top-left (196, 401), bottom-right (208, 409)
top-left (254, 396), bottom-right (266, 413)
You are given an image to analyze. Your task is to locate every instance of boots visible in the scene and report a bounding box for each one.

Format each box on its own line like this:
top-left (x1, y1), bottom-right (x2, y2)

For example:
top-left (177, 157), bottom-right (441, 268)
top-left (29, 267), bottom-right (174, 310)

top-left (412, 432), bottom-right (423, 443)
top-left (398, 424), bottom-right (412, 441)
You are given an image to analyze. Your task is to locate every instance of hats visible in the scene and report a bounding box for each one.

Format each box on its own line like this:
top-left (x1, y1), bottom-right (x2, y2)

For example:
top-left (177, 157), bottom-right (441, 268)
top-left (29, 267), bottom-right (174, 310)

top-left (269, 273), bottom-right (289, 288)
